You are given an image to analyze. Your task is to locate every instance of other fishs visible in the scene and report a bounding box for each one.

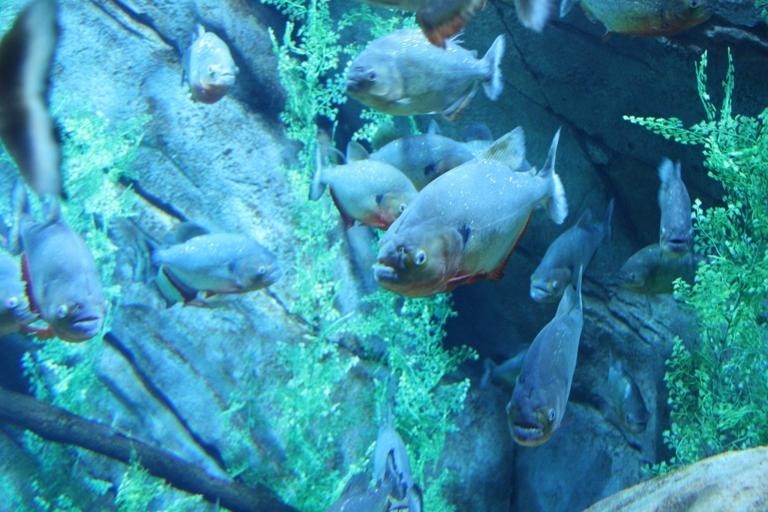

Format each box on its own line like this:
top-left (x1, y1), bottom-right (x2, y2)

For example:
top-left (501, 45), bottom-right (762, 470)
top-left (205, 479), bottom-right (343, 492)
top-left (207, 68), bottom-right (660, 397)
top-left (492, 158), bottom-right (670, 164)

top-left (559, 0), bottom-right (716, 42)
top-left (529, 199), bottom-right (615, 305)
top-left (0, 196), bottom-right (105, 343)
top-left (344, 27), bottom-right (506, 121)
top-left (371, 0), bottom-right (485, 50)
top-left (127, 218), bottom-right (284, 303)
top-left (615, 157), bottom-right (707, 294)
top-left (0, 1), bottom-right (68, 200)
top-left (310, 125), bottom-right (568, 298)
top-left (606, 348), bottom-right (652, 434)
top-left (177, 22), bottom-right (240, 104)
top-left (480, 265), bottom-right (584, 448)
